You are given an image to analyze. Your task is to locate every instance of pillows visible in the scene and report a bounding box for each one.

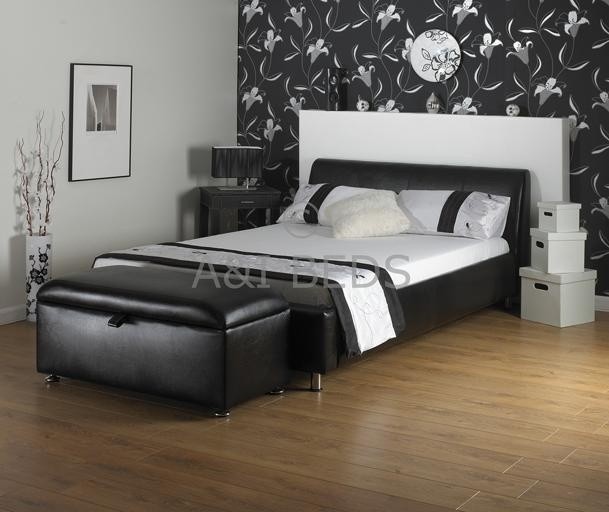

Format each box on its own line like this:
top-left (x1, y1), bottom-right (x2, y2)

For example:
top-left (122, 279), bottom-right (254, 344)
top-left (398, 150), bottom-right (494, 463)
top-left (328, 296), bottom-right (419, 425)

top-left (400, 189), bottom-right (511, 240)
top-left (278, 183), bottom-right (380, 225)
top-left (321, 190), bottom-right (410, 240)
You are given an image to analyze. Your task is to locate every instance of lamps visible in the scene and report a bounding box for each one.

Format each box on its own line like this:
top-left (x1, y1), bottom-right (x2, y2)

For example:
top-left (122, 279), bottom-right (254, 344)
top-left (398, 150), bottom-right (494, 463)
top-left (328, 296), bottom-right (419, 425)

top-left (211, 146), bottom-right (264, 190)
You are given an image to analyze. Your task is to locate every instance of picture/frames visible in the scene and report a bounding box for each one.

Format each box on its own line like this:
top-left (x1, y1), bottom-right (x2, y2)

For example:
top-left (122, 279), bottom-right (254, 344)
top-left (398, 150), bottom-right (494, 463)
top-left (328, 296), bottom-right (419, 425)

top-left (67, 63), bottom-right (132, 181)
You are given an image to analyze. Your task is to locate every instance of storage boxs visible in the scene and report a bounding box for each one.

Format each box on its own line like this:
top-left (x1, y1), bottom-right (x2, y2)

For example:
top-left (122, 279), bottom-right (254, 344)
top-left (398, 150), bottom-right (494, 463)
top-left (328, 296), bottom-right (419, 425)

top-left (530, 227), bottom-right (588, 275)
top-left (537, 201), bottom-right (581, 234)
top-left (519, 266), bottom-right (598, 329)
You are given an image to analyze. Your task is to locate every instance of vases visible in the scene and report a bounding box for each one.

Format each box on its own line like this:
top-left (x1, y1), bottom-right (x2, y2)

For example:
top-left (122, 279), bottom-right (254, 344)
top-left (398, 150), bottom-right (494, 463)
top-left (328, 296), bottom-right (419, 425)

top-left (25, 232), bottom-right (53, 322)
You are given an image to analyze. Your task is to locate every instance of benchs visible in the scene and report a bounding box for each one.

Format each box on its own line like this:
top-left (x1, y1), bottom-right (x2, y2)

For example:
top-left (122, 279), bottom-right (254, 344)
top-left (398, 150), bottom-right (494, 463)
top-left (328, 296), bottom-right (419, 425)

top-left (36, 266), bottom-right (291, 418)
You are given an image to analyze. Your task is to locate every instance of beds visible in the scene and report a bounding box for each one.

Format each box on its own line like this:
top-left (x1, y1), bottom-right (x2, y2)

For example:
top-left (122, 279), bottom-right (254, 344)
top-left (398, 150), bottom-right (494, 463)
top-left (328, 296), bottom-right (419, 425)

top-left (92, 160), bottom-right (532, 392)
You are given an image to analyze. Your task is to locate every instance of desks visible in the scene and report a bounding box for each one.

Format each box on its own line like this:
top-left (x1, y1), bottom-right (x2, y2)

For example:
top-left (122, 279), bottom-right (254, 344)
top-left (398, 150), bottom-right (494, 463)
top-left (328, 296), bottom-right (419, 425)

top-left (200, 184), bottom-right (281, 237)
